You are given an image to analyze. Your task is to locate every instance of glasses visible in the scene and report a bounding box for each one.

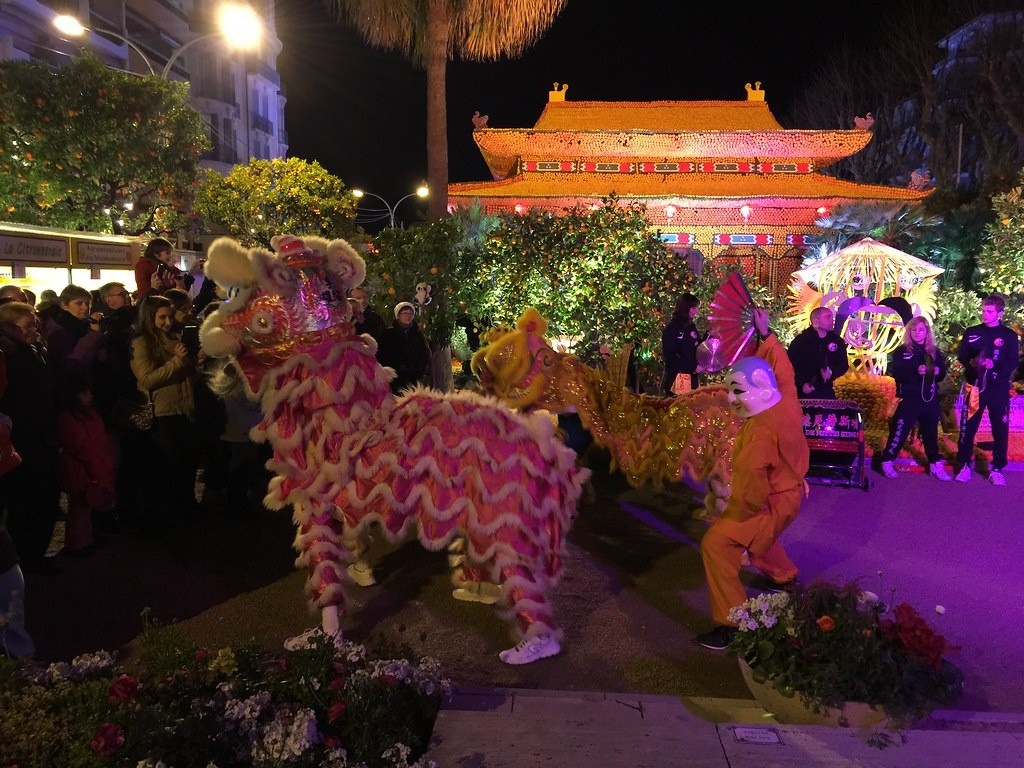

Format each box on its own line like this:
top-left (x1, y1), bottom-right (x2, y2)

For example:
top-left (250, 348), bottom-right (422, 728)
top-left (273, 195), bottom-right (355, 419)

top-left (400, 311), bottom-right (414, 317)
top-left (104, 292), bottom-right (130, 297)
top-left (176, 305), bottom-right (197, 313)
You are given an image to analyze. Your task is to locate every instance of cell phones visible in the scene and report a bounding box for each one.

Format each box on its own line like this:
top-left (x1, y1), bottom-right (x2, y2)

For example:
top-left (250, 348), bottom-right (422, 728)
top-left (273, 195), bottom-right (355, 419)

top-left (156, 264), bottom-right (164, 279)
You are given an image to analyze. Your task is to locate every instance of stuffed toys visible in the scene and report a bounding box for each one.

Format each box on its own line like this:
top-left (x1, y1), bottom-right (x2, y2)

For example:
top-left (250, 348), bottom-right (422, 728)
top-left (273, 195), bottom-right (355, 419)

top-left (469, 307), bottom-right (745, 528)
top-left (193, 238), bottom-right (591, 669)
top-left (414, 282), bottom-right (432, 306)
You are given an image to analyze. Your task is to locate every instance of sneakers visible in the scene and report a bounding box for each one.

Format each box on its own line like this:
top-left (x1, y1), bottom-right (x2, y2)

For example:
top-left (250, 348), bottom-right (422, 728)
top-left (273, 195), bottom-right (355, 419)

top-left (283, 624), bottom-right (346, 651)
top-left (764, 577), bottom-right (809, 600)
top-left (500, 633), bottom-right (560, 665)
top-left (452, 587), bottom-right (501, 604)
top-left (346, 562), bottom-right (376, 588)
top-left (695, 624), bottom-right (741, 651)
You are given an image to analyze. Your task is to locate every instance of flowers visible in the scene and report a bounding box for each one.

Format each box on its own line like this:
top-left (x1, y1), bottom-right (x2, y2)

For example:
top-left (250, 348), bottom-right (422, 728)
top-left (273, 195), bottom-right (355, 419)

top-left (723, 573), bottom-right (964, 751)
top-left (0, 603), bottom-right (461, 768)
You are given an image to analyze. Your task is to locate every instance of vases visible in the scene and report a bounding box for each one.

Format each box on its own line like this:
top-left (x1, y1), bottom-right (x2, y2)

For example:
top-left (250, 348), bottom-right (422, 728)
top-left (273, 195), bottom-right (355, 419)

top-left (738, 657), bottom-right (886, 729)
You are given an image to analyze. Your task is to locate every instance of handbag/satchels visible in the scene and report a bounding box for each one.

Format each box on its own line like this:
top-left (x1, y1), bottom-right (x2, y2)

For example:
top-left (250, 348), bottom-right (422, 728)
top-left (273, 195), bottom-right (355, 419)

top-left (119, 403), bottom-right (154, 431)
top-left (669, 373), bottom-right (691, 395)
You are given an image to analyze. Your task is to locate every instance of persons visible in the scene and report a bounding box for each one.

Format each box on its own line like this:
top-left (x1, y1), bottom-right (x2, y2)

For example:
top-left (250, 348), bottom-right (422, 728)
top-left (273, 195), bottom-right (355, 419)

top-left (788, 307), bottom-right (851, 400)
top-left (881, 315), bottom-right (952, 482)
top-left (660, 293), bottom-right (711, 399)
top-left (346, 285), bottom-right (388, 370)
top-left (697, 307), bottom-right (811, 649)
top-left (0, 258), bottom-right (274, 661)
top-left (954, 296), bottom-right (1019, 486)
top-left (132, 237), bottom-right (183, 296)
top-left (381, 302), bottom-right (431, 395)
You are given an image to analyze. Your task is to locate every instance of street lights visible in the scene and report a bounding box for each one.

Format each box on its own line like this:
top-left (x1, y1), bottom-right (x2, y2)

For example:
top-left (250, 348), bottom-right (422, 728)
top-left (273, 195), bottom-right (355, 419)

top-left (49, 5), bottom-right (264, 236)
top-left (353, 186), bottom-right (428, 258)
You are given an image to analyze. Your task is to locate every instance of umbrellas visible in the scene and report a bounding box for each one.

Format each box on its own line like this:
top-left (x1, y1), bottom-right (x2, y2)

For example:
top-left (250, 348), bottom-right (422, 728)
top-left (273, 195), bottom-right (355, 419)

top-left (790, 236), bottom-right (945, 283)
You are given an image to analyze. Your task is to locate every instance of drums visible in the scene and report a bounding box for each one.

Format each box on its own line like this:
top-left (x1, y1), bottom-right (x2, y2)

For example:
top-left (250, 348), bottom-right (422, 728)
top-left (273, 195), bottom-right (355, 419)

top-left (795, 398), bottom-right (861, 455)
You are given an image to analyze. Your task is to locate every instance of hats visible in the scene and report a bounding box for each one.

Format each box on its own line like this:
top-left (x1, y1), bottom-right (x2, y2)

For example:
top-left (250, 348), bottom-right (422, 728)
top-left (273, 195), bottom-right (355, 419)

top-left (394, 302), bottom-right (416, 319)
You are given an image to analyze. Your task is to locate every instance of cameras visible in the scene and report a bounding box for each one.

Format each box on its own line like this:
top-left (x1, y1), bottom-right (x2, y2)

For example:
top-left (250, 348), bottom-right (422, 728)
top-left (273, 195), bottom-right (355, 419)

top-left (99, 315), bottom-right (115, 333)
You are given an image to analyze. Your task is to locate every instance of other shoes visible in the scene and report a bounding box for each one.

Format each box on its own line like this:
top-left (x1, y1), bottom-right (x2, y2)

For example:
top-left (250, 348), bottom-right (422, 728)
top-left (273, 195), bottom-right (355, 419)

top-left (988, 470), bottom-right (1006, 486)
top-left (35, 654), bottom-right (58, 663)
top-left (880, 461), bottom-right (900, 479)
top-left (956, 464), bottom-right (973, 481)
top-left (930, 462), bottom-right (952, 481)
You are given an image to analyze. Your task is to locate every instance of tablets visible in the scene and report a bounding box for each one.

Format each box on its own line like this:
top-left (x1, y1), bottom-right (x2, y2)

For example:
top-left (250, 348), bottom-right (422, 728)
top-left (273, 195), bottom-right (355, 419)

top-left (181, 326), bottom-right (197, 358)
